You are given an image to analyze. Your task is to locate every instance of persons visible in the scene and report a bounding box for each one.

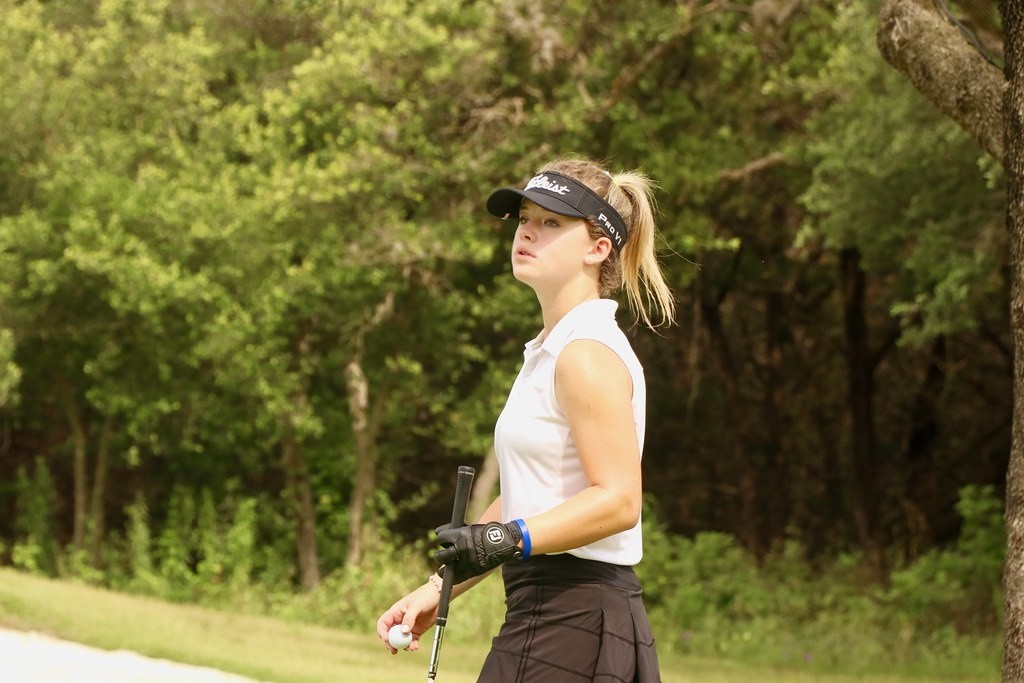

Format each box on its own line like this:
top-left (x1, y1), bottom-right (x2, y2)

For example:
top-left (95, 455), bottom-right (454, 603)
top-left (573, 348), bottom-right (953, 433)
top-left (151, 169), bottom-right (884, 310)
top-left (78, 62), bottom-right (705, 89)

top-left (378, 159), bottom-right (676, 683)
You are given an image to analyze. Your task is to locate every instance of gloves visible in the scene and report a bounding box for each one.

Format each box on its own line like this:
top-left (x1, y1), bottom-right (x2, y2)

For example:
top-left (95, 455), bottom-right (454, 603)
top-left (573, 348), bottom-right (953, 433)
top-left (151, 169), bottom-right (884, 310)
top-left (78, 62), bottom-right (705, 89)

top-left (434, 522), bottom-right (524, 585)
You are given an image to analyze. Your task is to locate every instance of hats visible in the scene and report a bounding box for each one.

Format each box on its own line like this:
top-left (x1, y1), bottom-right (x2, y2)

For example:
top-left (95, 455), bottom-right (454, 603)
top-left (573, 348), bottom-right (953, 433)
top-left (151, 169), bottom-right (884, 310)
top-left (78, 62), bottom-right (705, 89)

top-left (487, 171), bottom-right (627, 252)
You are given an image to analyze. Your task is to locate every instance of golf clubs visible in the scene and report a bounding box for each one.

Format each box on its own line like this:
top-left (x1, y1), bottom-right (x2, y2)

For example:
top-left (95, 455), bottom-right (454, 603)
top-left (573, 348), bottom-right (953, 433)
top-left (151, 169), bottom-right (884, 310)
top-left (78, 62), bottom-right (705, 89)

top-left (426, 464), bottom-right (476, 683)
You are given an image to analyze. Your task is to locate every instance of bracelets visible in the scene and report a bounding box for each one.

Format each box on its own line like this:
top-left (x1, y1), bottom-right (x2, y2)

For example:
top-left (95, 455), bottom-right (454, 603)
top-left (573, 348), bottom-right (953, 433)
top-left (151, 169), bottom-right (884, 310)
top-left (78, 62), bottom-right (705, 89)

top-left (515, 519), bottom-right (531, 560)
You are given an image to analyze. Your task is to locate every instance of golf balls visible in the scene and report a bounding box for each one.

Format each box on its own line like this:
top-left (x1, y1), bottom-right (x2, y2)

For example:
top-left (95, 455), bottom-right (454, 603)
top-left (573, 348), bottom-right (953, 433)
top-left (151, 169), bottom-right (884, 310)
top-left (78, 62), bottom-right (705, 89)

top-left (388, 625), bottom-right (413, 650)
